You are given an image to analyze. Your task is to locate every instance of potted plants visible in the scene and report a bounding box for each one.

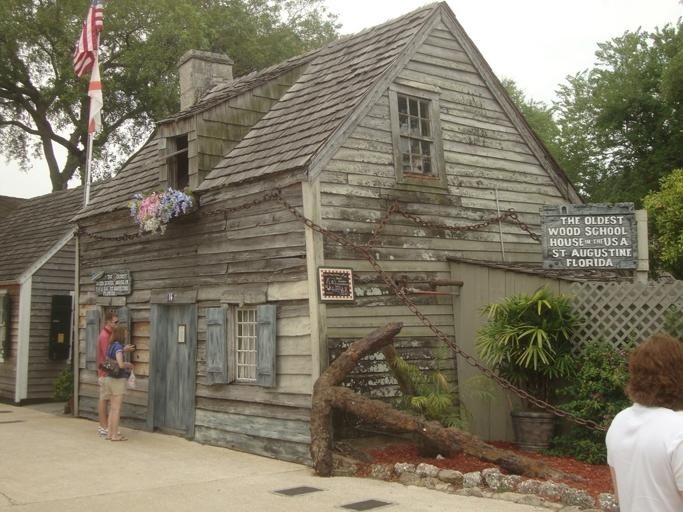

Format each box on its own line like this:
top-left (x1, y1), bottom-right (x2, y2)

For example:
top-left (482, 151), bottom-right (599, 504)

top-left (477, 288), bottom-right (578, 451)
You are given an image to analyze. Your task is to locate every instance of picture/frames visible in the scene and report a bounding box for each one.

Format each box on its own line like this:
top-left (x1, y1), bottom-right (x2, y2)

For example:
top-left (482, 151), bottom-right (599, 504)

top-left (318, 265), bottom-right (355, 304)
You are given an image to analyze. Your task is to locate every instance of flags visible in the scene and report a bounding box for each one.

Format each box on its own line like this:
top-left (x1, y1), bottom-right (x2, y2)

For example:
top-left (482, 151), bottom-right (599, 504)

top-left (72, 0), bottom-right (105, 134)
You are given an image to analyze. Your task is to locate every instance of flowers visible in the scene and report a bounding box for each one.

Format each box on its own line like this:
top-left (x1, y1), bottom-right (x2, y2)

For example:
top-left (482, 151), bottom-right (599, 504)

top-left (125, 190), bottom-right (194, 238)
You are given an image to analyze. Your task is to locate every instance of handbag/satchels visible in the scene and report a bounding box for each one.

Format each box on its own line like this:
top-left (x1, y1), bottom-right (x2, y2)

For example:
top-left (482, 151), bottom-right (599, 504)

top-left (99, 356), bottom-right (120, 377)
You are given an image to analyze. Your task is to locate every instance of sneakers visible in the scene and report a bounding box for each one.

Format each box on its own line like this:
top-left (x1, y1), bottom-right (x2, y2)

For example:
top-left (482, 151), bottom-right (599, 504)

top-left (97, 425), bottom-right (127, 441)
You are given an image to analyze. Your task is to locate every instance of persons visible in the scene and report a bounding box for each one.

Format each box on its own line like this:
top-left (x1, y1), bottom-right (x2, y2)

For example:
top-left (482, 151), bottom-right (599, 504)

top-left (96, 311), bottom-right (135, 435)
top-left (104, 325), bottom-right (134, 441)
top-left (605, 334), bottom-right (683, 512)
